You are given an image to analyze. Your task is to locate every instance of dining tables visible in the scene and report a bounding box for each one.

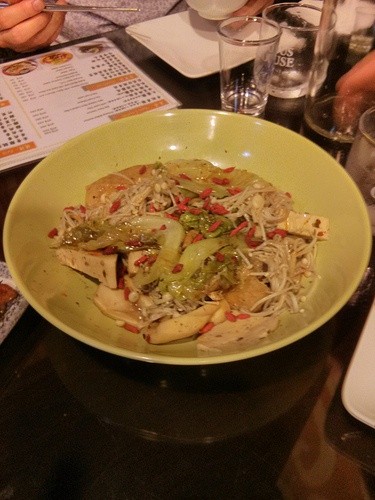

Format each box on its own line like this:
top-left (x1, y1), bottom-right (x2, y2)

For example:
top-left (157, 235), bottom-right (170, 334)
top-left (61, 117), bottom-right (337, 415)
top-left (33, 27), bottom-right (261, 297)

top-left (1, 27), bottom-right (374, 499)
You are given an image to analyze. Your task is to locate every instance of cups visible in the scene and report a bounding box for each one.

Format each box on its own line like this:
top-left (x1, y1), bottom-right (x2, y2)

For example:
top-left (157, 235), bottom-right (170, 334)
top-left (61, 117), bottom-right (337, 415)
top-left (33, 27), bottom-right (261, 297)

top-left (308, 91), bottom-right (366, 209)
top-left (258, 3), bottom-right (337, 100)
top-left (220, 17), bottom-right (279, 115)
top-left (345, 109), bottom-right (375, 205)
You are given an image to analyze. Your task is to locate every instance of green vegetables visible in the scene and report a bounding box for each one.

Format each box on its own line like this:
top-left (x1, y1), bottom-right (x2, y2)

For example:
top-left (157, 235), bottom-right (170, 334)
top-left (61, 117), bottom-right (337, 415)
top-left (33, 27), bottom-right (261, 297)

top-left (63, 206), bottom-right (259, 305)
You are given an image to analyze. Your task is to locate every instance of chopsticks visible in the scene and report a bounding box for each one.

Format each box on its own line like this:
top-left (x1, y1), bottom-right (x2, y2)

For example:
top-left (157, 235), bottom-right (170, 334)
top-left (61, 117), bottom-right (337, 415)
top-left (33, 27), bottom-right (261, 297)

top-left (1, 0), bottom-right (142, 14)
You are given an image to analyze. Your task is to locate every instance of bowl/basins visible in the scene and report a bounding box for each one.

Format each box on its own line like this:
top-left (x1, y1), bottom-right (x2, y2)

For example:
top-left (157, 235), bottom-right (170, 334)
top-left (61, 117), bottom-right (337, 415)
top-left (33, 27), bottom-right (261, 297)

top-left (185, 0), bottom-right (248, 20)
top-left (3, 108), bottom-right (373, 367)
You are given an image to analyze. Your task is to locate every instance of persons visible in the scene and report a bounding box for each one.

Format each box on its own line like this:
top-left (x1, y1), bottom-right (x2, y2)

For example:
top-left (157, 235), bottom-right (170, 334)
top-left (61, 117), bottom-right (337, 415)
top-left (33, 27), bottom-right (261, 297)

top-left (1, 0), bottom-right (276, 54)
top-left (333, 49), bottom-right (375, 127)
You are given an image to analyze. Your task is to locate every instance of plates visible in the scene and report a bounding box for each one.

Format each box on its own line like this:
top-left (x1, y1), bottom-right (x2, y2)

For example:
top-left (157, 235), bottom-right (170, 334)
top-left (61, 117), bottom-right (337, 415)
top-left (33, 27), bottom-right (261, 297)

top-left (342, 298), bottom-right (375, 430)
top-left (122, 8), bottom-right (311, 79)
